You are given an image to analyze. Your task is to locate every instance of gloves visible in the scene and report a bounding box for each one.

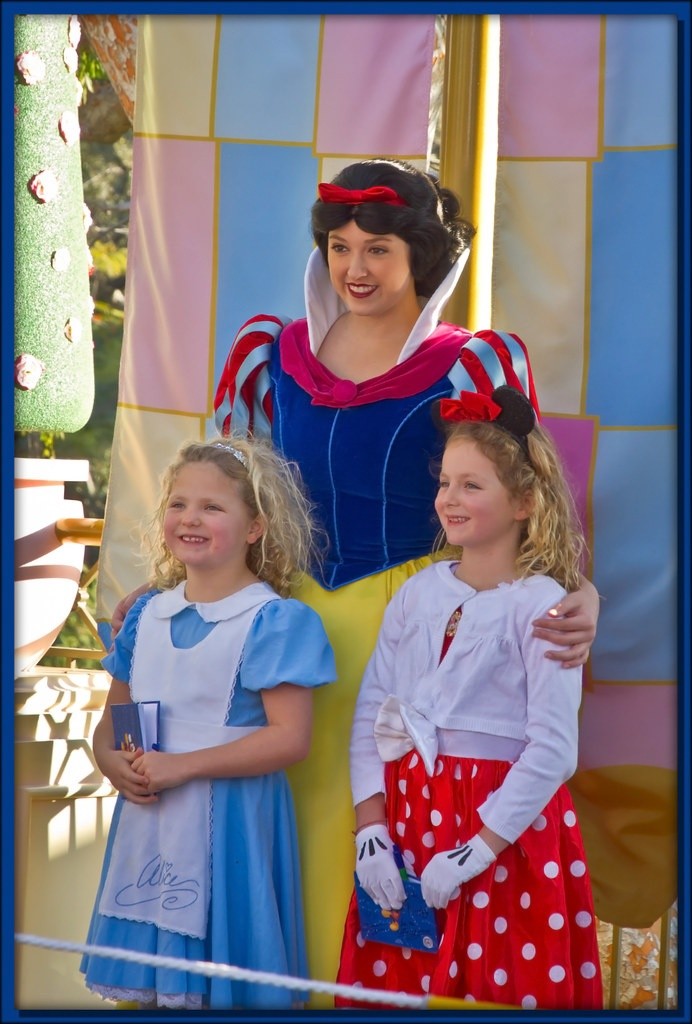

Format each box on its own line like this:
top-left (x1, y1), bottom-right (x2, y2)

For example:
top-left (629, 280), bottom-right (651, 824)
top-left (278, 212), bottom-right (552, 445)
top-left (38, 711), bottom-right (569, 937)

top-left (355, 824), bottom-right (407, 910)
top-left (422, 837), bottom-right (497, 909)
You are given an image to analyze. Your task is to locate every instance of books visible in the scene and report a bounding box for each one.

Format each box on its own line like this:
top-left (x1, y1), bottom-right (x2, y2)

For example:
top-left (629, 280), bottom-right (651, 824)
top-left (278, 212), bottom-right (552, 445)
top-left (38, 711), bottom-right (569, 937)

top-left (353, 870), bottom-right (437, 953)
top-left (111, 701), bottom-right (162, 797)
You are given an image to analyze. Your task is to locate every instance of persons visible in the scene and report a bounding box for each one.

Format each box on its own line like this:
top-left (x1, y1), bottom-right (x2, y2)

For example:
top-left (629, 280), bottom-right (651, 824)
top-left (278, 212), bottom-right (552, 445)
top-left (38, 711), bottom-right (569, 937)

top-left (79, 427), bottom-right (337, 1010)
top-left (333, 385), bottom-right (603, 1010)
top-left (110, 159), bottom-right (601, 1018)
